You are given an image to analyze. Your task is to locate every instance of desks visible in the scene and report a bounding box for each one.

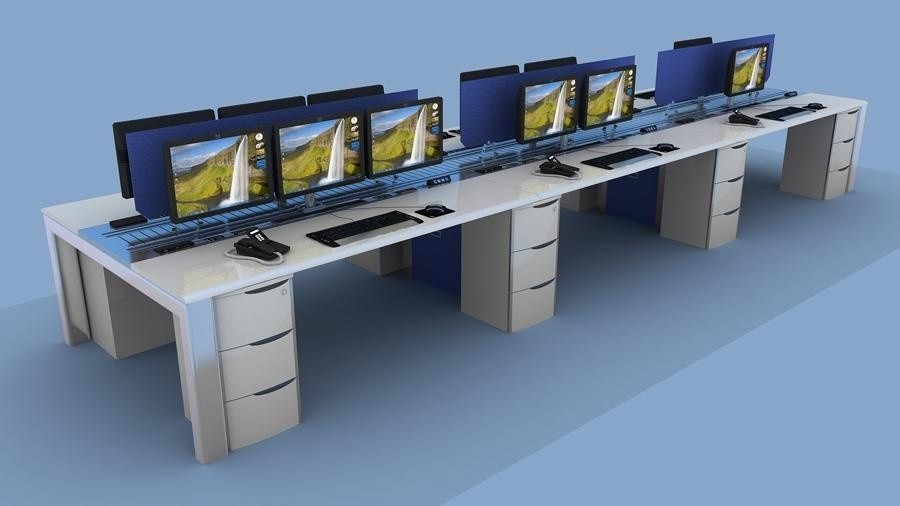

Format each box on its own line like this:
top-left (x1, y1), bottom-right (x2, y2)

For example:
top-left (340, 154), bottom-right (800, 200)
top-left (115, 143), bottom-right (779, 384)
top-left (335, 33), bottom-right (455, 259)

top-left (39, 81), bottom-right (868, 463)
